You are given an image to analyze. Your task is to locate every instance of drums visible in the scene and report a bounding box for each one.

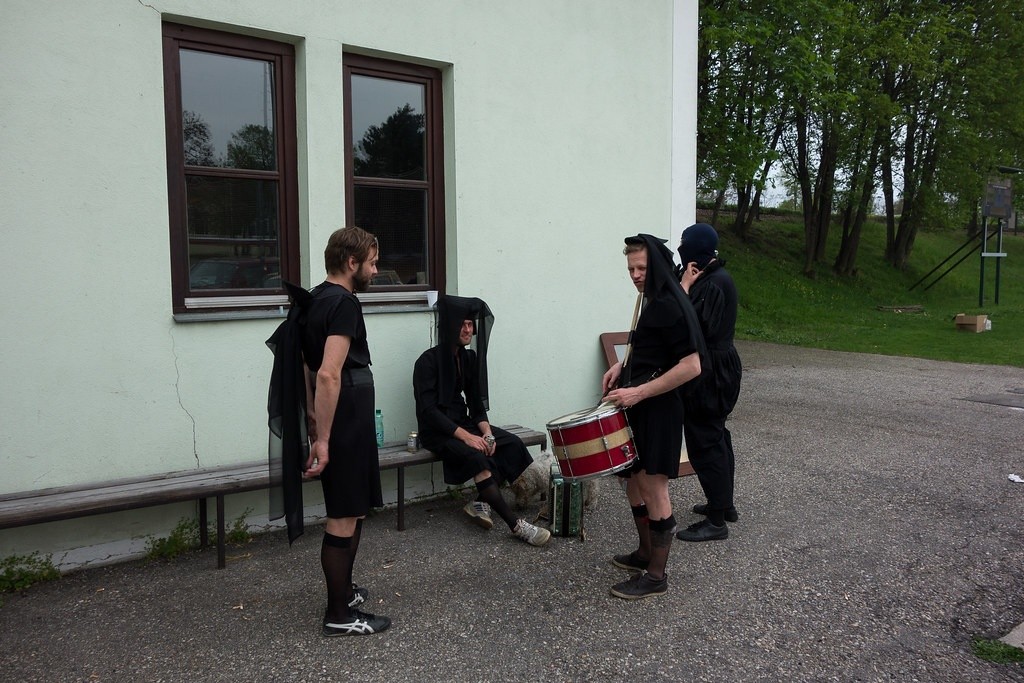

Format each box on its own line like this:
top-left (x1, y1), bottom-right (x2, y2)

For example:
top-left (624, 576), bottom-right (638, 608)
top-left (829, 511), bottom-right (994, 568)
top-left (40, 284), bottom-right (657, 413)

top-left (545, 405), bottom-right (640, 487)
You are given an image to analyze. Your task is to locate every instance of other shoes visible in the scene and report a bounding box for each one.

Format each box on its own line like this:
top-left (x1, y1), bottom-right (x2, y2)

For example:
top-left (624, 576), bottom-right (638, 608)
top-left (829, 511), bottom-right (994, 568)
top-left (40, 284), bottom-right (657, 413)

top-left (676, 518), bottom-right (728, 542)
top-left (693, 504), bottom-right (738, 522)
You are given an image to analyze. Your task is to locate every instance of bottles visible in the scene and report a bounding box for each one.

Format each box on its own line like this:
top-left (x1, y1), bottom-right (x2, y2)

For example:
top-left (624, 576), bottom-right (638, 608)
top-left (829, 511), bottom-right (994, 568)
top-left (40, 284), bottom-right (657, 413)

top-left (375, 409), bottom-right (384, 447)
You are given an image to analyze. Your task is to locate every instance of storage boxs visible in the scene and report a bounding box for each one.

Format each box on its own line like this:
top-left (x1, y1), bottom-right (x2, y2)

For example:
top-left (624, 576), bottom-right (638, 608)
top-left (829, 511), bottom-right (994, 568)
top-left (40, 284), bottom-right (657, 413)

top-left (952, 313), bottom-right (988, 333)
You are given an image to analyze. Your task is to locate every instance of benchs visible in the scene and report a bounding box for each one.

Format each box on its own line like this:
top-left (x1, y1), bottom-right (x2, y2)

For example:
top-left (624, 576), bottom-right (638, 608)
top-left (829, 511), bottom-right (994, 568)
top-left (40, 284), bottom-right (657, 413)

top-left (2, 424), bottom-right (547, 569)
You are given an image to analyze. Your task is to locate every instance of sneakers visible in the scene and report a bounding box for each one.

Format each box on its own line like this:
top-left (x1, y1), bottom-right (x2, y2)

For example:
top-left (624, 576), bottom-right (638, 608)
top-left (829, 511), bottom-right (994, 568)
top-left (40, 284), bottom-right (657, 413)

top-left (340, 588), bottom-right (368, 610)
top-left (463, 500), bottom-right (494, 529)
top-left (610, 570), bottom-right (669, 598)
top-left (322, 607), bottom-right (391, 637)
top-left (511, 519), bottom-right (550, 546)
top-left (612, 549), bottom-right (649, 570)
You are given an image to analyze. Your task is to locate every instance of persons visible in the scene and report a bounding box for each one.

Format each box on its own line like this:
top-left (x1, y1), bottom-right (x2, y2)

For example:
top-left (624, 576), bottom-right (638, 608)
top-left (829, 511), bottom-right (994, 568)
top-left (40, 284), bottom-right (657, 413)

top-left (415, 296), bottom-right (552, 548)
top-left (600, 233), bottom-right (712, 601)
top-left (672, 225), bottom-right (744, 544)
top-left (297, 228), bottom-right (394, 639)
